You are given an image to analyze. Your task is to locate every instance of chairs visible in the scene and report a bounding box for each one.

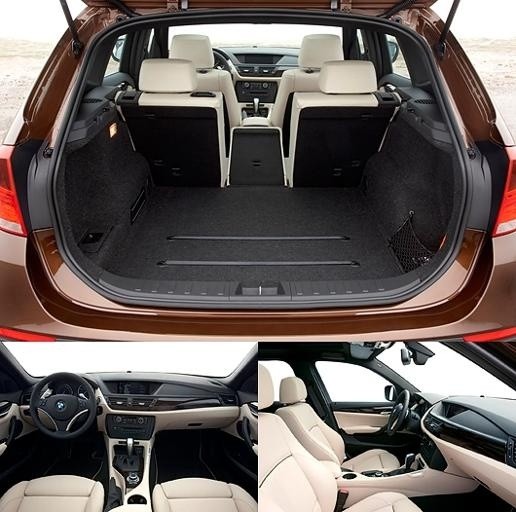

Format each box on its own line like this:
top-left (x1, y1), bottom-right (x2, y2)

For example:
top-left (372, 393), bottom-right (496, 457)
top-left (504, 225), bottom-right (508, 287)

top-left (269, 33), bottom-right (345, 128)
top-left (0, 474), bottom-right (105, 512)
top-left (138, 59), bottom-right (230, 189)
top-left (280, 58), bottom-right (403, 189)
top-left (152, 478), bottom-right (258, 512)
top-left (274, 376), bottom-right (401, 480)
top-left (168, 34), bottom-right (241, 128)
top-left (258, 363), bottom-right (423, 512)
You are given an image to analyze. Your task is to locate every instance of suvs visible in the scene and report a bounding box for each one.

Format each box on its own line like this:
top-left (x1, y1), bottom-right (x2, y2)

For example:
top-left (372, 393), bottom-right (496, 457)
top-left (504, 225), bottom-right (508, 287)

top-left (0, 0), bottom-right (516, 342)
